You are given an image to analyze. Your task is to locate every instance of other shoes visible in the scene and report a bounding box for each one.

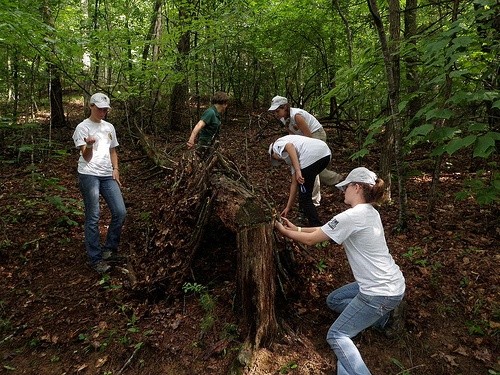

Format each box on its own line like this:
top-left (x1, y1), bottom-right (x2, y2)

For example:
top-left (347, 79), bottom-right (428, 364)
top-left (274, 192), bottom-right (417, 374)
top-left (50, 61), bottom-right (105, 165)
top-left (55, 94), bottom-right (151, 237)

top-left (94, 259), bottom-right (111, 276)
top-left (385, 301), bottom-right (408, 338)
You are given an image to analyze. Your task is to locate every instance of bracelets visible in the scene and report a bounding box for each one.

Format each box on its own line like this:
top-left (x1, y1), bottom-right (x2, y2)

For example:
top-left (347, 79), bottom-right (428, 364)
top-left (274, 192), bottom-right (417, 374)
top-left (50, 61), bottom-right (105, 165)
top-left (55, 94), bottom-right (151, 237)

top-left (114, 168), bottom-right (119, 171)
top-left (297, 226), bottom-right (302, 232)
top-left (86, 144), bottom-right (93, 148)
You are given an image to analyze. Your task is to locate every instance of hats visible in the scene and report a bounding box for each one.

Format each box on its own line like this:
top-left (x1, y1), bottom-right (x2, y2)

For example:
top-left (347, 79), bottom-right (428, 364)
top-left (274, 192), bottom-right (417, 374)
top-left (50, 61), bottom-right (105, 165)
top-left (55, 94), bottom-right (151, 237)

top-left (268, 95), bottom-right (287, 112)
top-left (90, 93), bottom-right (112, 109)
top-left (268, 142), bottom-right (273, 161)
top-left (335, 167), bottom-right (377, 192)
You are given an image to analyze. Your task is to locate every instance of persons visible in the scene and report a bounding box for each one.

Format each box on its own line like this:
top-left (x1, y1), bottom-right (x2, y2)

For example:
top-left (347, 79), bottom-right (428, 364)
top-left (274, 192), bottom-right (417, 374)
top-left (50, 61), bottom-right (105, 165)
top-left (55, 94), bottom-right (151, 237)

top-left (274, 167), bottom-right (406, 375)
top-left (184, 92), bottom-right (229, 159)
top-left (268, 95), bottom-right (344, 207)
top-left (268, 134), bottom-right (332, 227)
top-left (72, 93), bottom-right (126, 273)
